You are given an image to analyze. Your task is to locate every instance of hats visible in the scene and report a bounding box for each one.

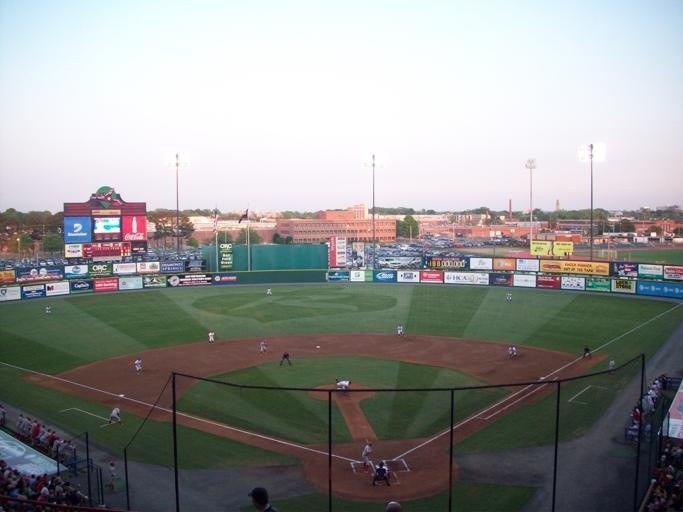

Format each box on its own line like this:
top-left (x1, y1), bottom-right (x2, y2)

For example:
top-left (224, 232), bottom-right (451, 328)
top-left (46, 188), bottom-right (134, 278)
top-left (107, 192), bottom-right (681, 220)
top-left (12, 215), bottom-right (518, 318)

top-left (248, 488), bottom-right (267, 498)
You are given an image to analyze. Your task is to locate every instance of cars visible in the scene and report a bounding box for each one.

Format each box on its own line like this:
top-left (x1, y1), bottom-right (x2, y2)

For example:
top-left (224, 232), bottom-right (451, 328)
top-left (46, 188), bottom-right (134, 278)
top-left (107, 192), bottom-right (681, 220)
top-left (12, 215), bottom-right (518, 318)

top-left (0, 247), bottom-right (202, 270)
top-left (414, 235), bottom-right (527, 250)
top-left (325, 244), bottom-right (482, 270)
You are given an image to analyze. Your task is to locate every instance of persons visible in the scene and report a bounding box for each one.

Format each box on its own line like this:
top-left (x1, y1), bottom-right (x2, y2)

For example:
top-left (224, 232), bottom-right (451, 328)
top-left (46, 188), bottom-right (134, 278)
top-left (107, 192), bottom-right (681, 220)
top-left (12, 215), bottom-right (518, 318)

top-left (508, 346), bottom-right (512, 357)
top-left (396, 323), bottom-right (402, 335)
top-left (247, 486), bottom-right (277, 512)
top-left (265, 288), bottom-right (272, 296)
top-left (0, 403), bottom-right (124, 512)
top-left (505, 291), bottom-right (512, 301)
top-left (259, 339), bottom-right (267, 352)
top-left (334, 380), bottom-right (351, 391)
top-left (622, 374), bottom-right (681, 512)
top-left (607, 358), bottom-right (616, 370)
top-left (370, 462), bottom-right (388, 487)
top-left (207, 330), bottom-right (215, 343)
top-left (134, 357), bottom-right (142, 371)
top-left (278, 352), bottom-right (291, 367)
top-left (44, 305), bottom-right (51, 314)
top-left (511, 345), bottom-right (516, 356)
top-left (361, 441), bottom-right (372, 472)
top-left (581, 345), bottom-right (592, 359)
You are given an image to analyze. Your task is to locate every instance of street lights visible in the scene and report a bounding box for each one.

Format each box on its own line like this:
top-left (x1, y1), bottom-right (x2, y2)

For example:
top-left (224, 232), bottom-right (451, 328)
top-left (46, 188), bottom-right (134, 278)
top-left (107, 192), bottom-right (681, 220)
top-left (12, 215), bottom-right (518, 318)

top-left (524, 159), bottom-right (537, 255)
top-left (15, 238), bottom-right (20, 261)
top-left (587, 144), bottom-right (595, 259)
top-left (370, 153), bottom-right (376, 269)
top-left (175, 153), bottom-right (179, 255)
